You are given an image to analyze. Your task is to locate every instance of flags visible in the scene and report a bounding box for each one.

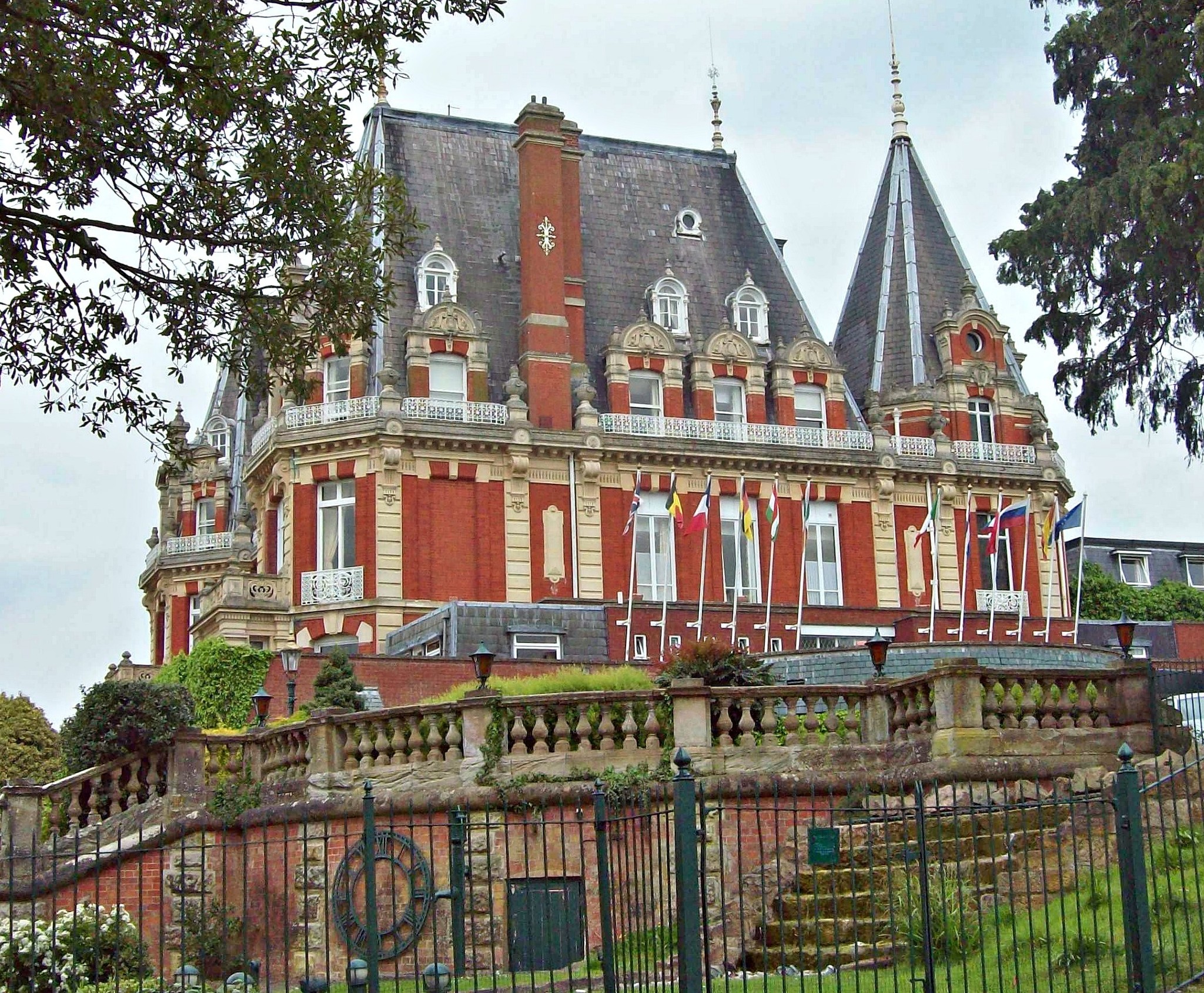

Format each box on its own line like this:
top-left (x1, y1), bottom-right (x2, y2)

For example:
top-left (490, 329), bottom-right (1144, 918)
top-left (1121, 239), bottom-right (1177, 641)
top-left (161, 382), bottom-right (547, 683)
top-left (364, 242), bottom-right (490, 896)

top-left (976, 509), bottom-right (999, 557)
top-left (965, 494), bottom-right (971, 555)
top-left (764, 480), bottom-right (780, 544)
top-left (665, 472), bottom-right (684, 529)
top-left (622, 469), bottom-right (641, 536)
top-left (683, 477), bottom-right (712, 537)
top-left (801, 480), bottom-right (810, 540)
top-left (1048, 502), bottom-right (1083, 553)
top-left (1040, 501), bottom-right (1056, 561)
top-left (914, 491), bottom-right (941, 547)
top-left (739, 477), bottom-right (753, 541)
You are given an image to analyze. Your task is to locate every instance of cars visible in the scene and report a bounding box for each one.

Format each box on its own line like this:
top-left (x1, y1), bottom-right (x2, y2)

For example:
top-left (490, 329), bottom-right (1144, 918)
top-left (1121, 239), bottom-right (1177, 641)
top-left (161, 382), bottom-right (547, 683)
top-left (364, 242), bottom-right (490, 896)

top-left (1160, 692), bottom-right (1204, 741)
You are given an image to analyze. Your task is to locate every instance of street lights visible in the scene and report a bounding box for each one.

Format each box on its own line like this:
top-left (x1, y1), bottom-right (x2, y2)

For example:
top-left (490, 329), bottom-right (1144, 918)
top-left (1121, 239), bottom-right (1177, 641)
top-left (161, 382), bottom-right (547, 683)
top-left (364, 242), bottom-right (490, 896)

top-left (281, 630), bottom-right (304, 717)
